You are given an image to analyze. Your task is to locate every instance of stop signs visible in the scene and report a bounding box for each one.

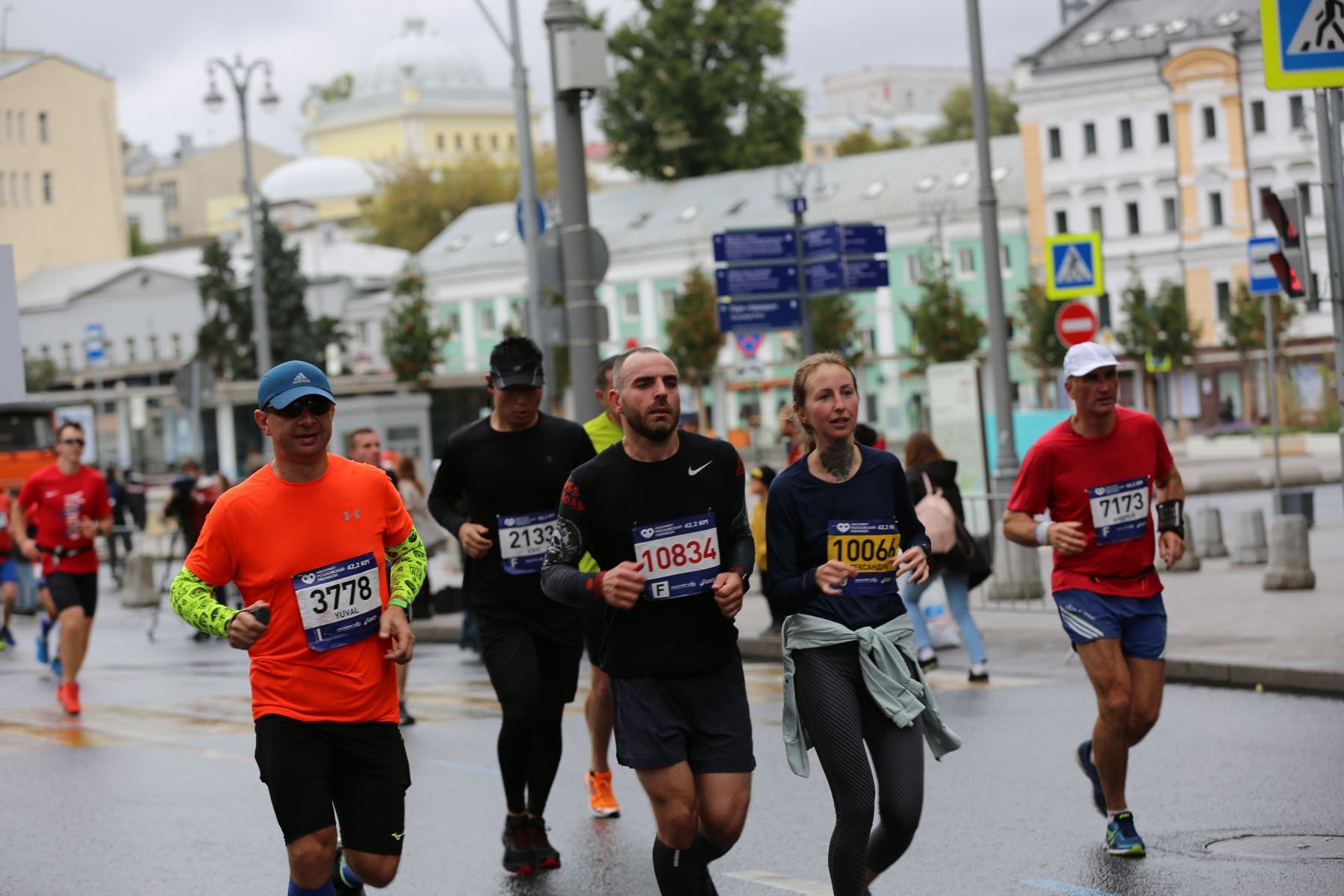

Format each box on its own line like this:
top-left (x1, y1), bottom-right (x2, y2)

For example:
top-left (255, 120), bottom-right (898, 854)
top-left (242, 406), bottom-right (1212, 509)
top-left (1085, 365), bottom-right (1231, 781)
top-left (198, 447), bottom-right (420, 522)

top-left (1054, 301), bottom-right (1098, 350)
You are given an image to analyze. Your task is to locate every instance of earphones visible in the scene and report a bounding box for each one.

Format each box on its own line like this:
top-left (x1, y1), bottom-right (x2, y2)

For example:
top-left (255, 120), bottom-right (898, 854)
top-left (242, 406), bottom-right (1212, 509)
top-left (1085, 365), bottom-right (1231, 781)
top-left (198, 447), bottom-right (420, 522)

top-left (487, 382), bottom-right (492, 386)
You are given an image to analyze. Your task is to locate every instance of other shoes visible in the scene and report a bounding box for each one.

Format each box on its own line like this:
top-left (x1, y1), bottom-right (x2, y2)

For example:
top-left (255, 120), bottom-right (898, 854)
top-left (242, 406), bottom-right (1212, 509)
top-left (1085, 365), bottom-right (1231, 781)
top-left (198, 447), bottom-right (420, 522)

top-left (56, 681), bottom-right (82, 714)
top-left (50, 657), bottom-right (61, 674)
top-left (400, 699), bottom-right (415, 725)
top-left (334, 840), bottom-right (366, 896)
top-left (192, 630), bottom-right (210, 641)
top-left (917, 657), bottom-right (938, 674)
top-left (36, 636), bottom-right (48, 663)
top-left (968, 669), bottom-right (988, 683)
top-left (0, 626), bottom-right (15, 645)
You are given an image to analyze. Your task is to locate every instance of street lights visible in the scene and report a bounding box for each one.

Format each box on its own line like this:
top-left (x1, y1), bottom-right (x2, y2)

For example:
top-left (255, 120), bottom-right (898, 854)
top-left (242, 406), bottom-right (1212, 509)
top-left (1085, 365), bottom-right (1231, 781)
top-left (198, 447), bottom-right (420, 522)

top-left (205, 55), bottom-right (282, 463)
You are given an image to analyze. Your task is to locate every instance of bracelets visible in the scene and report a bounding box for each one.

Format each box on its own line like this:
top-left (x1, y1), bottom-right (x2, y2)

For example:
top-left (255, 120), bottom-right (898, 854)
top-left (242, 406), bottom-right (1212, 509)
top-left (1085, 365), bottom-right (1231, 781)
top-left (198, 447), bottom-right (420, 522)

top-left (1037, 521), bottom-right (1058, 547)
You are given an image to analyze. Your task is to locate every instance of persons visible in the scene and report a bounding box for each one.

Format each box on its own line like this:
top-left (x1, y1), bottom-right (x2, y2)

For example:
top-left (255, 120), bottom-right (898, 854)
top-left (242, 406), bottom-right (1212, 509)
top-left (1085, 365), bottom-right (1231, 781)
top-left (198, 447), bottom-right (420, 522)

top-left (0, 335), bottom-right (992, 896)
top-left (1002, 342), bottom-right (1186, 856)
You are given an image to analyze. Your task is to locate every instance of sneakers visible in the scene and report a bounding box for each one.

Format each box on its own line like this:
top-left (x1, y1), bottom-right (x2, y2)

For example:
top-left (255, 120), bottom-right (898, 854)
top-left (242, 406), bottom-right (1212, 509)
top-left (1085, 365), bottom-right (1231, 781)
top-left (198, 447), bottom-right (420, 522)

top-left (1075, 740), bottom-right (1108, 818)
top-left (502, 813), bottom-right (561, 875)
top-left (1102, 811), bottom-right (1146, 856)
top-left (587, 768), bottom-right (622, 819)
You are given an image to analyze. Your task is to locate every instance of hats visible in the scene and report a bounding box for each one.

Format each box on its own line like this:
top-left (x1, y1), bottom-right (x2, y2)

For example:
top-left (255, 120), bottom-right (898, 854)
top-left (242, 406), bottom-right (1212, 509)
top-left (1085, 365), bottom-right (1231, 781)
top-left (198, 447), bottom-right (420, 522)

top-left (257, 360), bottom-right (336, 412)
top-left (490, 360), bottom-right (545, 390)
top-left (1062, 340), bottom-right (1122, 379)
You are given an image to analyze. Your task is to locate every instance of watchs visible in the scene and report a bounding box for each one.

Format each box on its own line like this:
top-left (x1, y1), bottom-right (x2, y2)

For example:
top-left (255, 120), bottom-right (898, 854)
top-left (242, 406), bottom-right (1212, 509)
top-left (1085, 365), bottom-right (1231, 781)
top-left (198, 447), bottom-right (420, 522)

top-left (919, 541), bottom-right (932, 563)
top-left (387, 598), bottom-right (414, 624)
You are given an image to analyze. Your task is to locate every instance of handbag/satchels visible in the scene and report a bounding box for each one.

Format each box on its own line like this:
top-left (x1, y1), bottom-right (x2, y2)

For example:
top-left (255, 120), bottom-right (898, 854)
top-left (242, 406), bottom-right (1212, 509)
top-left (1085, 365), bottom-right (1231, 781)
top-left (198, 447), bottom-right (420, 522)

top-left (967, 542), bottom-right (992, 592)
top-left (913, 471), bottom-right (956, 554)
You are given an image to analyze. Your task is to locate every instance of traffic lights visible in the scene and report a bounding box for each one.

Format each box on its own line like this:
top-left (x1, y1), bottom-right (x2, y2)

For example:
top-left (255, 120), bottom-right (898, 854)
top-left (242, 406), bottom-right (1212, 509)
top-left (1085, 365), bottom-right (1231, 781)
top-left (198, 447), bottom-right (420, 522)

top-left (1257, 181), bottom-right (1315, 308)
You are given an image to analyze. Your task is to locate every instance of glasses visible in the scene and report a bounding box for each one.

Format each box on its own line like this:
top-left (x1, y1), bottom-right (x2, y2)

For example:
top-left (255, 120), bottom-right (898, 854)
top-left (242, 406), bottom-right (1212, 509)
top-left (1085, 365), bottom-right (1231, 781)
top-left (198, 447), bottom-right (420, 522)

top-left (267, 398), bottom-right (332, 419)
top-left (62, 438), bottom-right (84, 446)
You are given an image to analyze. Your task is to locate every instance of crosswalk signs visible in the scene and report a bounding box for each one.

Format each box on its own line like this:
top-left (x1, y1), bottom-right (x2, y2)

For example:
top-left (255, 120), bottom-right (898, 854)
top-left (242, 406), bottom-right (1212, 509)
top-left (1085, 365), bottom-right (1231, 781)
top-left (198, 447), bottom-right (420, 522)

top-left (1259, 0), bottom-right (1344, 90)
top-left (1043, 229), bottom-right (1105, 301)
